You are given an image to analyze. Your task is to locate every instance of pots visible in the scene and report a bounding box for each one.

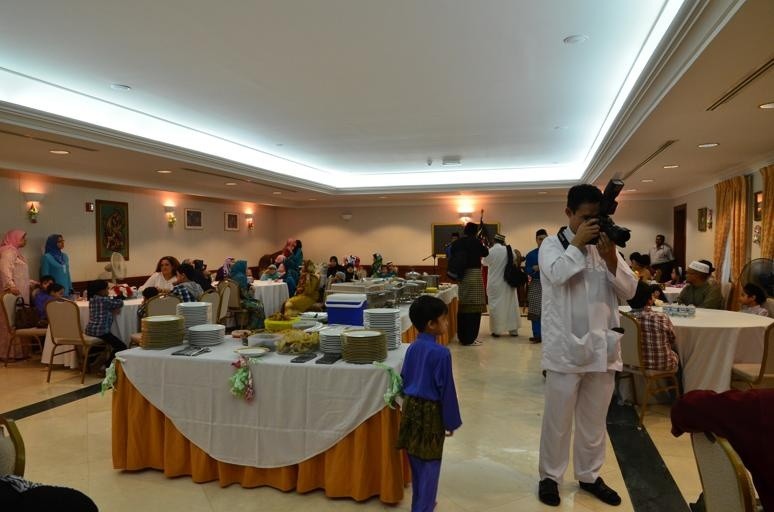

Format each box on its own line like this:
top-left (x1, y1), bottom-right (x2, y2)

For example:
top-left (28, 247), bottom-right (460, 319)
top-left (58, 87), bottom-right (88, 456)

top-left (419, 275), bottom-right (439, 288)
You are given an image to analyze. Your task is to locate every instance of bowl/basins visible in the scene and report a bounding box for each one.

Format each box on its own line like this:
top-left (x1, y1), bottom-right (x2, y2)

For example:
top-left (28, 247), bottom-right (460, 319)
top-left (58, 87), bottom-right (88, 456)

top-left (247, 316), bottom-right (322, 352)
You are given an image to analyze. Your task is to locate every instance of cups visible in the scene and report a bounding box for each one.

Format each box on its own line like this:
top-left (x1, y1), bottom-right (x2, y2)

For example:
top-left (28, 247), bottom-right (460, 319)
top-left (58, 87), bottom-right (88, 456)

top-left (662, 303), bottom-right (697, 317)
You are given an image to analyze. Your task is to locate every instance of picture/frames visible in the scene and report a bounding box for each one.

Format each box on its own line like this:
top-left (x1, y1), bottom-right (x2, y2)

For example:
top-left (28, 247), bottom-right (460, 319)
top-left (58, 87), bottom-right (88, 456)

top-left (184, 208), bottom-right (203, 229)
top-left (224, 212), bottom-right (239, 231)
top-left (95, 199), bottom-right (129, 261)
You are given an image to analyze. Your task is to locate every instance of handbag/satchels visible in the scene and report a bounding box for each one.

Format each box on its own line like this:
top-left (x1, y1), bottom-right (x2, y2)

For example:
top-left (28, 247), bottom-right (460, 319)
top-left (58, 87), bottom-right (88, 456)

top-left (14, 296), bottom-right (40, 328)
top-left (503, 263), bottom-right (526, 287)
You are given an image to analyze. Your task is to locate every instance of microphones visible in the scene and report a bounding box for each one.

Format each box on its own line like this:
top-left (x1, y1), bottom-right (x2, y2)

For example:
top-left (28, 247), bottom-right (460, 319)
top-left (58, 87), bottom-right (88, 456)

top-left (450, 239), bottom-right (456, 243)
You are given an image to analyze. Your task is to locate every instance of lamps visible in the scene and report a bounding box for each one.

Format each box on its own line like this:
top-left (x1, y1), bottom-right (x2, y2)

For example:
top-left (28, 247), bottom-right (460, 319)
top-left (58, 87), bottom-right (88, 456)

top-left (458, 212), bottom-right (472, 218)
top-left (341, 214), bottom-right (352, 221)
top-left (245, 214), bottom-right (253, 219)
top-left (164, 206), bottom-right (176, 213)
top-left (754, 191), bottom-right (763, 220)
top-left (23, 192), bottom-right (44, 203)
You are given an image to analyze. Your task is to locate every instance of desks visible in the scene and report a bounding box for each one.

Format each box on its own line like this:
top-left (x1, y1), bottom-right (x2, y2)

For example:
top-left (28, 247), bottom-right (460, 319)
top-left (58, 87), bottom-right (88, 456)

top-left (302, 284), bottom-right (459, 346)
top-left (112, 334), bottom-right (412, 504)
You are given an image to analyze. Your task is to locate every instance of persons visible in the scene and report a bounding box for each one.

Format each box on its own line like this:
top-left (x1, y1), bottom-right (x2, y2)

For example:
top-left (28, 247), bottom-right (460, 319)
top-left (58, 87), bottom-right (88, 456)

top-left (215, 257), bottom-right (266, 329)
top-left (393, 293), bottom-right (462, 512)
top-left (321, 253), bottom-right (397, 309)
top-left (537, 183), bottom-right (641, 508)
top-left (445, 220), bottom-right (549, 346)
top-left (617, 233), bottom-right (770, 404)
top-left (0, 228), bottom-right (32, 362)
top-left (137, 256), bottom-right (213, 318)
top-left (259, 238), bottom-right (320, 316)
top-left (34, 232), bottom-right (76, 329)
top-left (84, 281), bottom-right (128, 375)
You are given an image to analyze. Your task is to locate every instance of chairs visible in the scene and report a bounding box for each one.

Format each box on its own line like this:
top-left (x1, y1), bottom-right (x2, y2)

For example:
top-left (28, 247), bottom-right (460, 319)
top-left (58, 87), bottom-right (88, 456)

top-left (761, 297), bottom-right (774, 319)
top-left (618, 310), bottom-right (679, 430)
top-left (730, 322), bottom-right (774, 390)
top-left (0, 416), bottom-right (25, 477)
top-left (690, 430), bottom-right (757, 512)
top-left (720, 283), bottom-right (735, 310)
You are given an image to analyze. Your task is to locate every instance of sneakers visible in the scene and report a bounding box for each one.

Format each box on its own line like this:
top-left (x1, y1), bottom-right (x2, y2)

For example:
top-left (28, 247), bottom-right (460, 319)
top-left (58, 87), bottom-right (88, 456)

top-left (473, 339), bottom-right (482, 345)
top-left (529, 337), bottom-right (541, 345)
top-left (579, 476), bottom-right (621, 506)
top-left (539, 479), bottom-right (560, 506)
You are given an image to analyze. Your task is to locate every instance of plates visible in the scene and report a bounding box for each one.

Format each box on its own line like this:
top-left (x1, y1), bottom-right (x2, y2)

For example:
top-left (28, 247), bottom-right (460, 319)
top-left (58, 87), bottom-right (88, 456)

top-left (233, 345), bottom-right (270, 358)
top-left (319, 307), bottom-right (403, 364)
top-left (133, 300), bottom-right (228, 351)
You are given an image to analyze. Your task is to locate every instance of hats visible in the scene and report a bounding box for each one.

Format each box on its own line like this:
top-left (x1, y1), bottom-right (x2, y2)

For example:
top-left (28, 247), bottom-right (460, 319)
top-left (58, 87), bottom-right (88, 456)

top-left (689, 260), bottom-right (710, 274)
top-left (494, 233), bottom-right (506, 241)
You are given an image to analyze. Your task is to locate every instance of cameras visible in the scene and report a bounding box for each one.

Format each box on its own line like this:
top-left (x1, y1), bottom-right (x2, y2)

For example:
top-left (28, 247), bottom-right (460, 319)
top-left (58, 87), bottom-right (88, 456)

top-left (584, 176), bottom-right (632, 250)
